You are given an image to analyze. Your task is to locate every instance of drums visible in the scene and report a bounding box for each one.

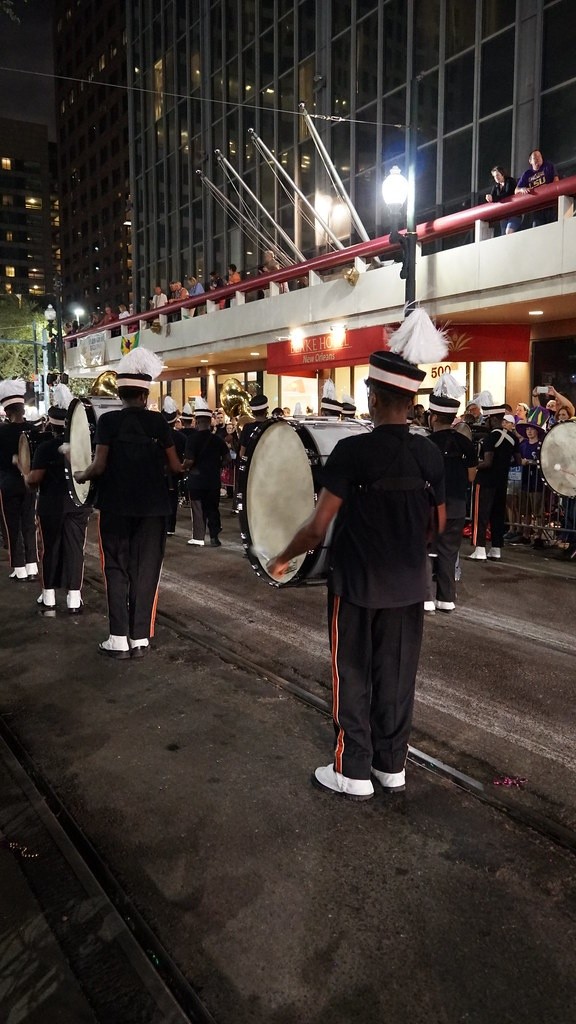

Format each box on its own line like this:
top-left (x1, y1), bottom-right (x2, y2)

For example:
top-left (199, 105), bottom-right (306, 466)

top-left (536, 418), bottom-right (575, 499)
top-left (408, 423), bottom-right (430, 438)
top-left (64, 394), bottom-right (123, 509)
top-left (177, 478), bottom-right (188, 505)
top-left (18, 431), bottom-right (53, 476)
top-left (452, 422), bottom-right (491, 467)
top-left (219, 453), bottom-right (235, 487)
top-left (235, 417), bottom-right (375, 589)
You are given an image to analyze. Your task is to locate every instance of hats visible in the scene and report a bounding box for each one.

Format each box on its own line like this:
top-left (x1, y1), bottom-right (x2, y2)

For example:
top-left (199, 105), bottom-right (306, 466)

top-left (318, 380), bottom-right (343, 414)
top-left (341, 394), bottom-right (356, 415)
top-left (48, 385), bottom-right (72, 425)
top-left (249, 395), bottom-right (269, 410)
top-left (428, 373), bottom-right (465, 416)
top-left (116, 347), bottom-right (164, 393)
top-left (160, 396), bottom-right (213, 424)
top-left (366, 307), bottom-right (448, 397)
top-left (0, 377), bottom-right (26, 411)
top-left (465, 391), bottom-right (551, 434)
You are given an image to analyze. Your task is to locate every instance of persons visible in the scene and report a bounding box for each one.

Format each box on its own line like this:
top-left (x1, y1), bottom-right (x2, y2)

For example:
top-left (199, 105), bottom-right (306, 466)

top-left (207, 272), bottom-right (227, 310)
top-left (514, 150), bottom-right (559, 228)
top-left (306, 385), bottom-right (576, 615)
top-left (485, 166), bottom-right (525, 235)
top-left (266, 350), bottom-right (447, 802)
top-left (259, 251), bottom-right (279, 290)
top-left (222, 264), bottom-right (244, 291)
top-left (0, 372), bottom-right (290, 659)
top-left (149, 281), bottom-right (195, 329)
top-left (188, 277), bottom-right (206, 316)
top-left (51, 303), bottom-right (138, 350)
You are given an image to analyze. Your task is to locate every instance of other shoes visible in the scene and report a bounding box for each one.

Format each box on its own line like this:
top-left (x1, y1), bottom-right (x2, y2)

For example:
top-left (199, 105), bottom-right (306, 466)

top-left (371, 765), bottom-right (407, 793)
top-left (211, 538), bottom-right (222, 547)
top-left (9, 562), bottom-right (40, 583)
top-left (464, 546), bottom-right (488, 560)
top-left (187, 538), bottom-right (205, 546)
top-left (65, 590), bottom-right (83, 612)
top-left (37, 589), bottom-right (56, 617)
top-left (314, 763), bottom-right (374, 801)
top-left (503, 531), bottom-right (569, 560)
top-left (424, 600), bottom-right (436, 611)
top-left (487, 547), bottom-right (501, 559)
top-left (129, 637), bottom-right (151, 659)
top-left (97, 635), bottom-right (131, 660)
top-left (434, 600), bottom-right (455, 610)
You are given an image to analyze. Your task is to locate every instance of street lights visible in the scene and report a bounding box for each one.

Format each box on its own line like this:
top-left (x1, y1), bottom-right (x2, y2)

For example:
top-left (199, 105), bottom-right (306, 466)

top-left (372, 76), bottom-right (420, 317)
top-left (44, 284), bottom-right (86, 373)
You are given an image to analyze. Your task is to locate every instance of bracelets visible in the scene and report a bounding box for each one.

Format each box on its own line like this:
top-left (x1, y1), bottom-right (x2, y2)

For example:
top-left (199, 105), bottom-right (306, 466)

top-left (532, 393), bottom-right (538, 397)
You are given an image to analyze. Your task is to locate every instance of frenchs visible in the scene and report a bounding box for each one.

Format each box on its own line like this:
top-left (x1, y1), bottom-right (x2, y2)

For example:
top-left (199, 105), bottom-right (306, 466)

top-left (87, 371), bottom-right (120, 399)
top-left (219, 378), bottom-right (256, 438)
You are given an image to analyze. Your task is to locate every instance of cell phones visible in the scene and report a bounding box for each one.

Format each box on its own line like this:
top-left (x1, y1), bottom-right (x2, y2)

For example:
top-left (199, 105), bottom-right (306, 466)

top-left (536, 387), bottom-right (549, 393)
